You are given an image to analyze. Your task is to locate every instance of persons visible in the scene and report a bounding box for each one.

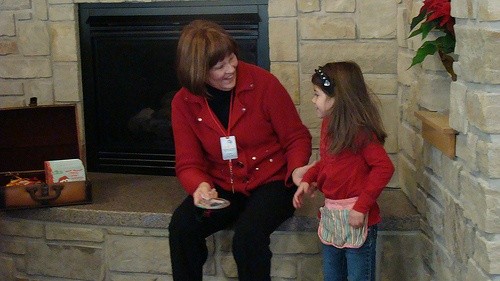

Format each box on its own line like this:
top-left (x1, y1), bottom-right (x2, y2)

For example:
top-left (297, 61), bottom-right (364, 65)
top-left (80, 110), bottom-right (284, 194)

top-left (165, 19), bottom-right (320, 281)
top-left (292, 61), bottom-right (397, 281)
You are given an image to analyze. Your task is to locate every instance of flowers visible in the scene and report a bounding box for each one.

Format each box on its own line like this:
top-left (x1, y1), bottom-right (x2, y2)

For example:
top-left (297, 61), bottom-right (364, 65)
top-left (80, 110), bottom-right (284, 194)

top-left (407, 0), bottom-right (458, 73)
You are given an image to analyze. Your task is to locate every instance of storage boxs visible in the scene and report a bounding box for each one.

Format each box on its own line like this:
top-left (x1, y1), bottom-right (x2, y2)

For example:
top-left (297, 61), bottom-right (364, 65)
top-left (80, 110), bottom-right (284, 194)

top-left (0, 97), bottom-right (91, 208)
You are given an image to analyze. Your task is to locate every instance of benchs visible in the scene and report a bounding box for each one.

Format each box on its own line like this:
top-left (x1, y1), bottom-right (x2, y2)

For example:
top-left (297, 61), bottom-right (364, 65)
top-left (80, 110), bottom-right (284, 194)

top-left (0, 173), bottom-right (421, 281)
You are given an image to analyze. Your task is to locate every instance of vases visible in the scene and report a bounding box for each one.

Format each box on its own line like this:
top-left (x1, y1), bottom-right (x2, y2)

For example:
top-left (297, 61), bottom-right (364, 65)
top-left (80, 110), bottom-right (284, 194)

top-left (415, 112), bottom-right (458, 158)
